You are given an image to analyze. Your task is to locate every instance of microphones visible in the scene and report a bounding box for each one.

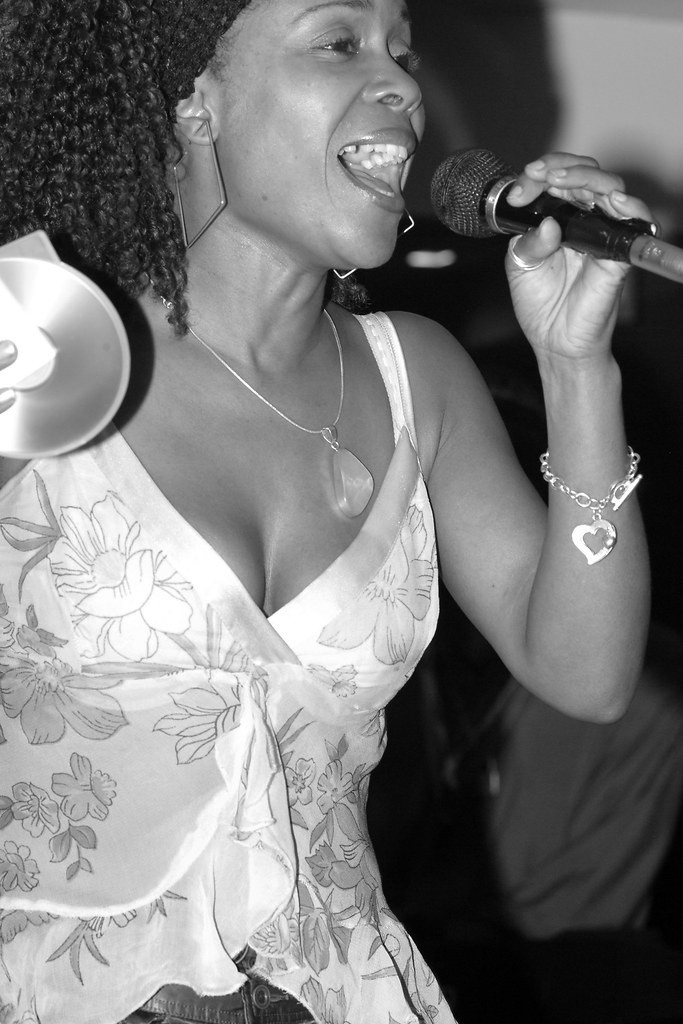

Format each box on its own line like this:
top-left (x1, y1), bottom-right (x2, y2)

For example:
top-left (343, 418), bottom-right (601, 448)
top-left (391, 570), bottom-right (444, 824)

top-left (430, 149), bottom-right (683, 283)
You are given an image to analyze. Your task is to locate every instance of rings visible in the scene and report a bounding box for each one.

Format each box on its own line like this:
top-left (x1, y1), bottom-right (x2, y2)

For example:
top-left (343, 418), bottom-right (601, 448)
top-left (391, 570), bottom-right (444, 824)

top-left (506, 233), bottom-right (544, 272)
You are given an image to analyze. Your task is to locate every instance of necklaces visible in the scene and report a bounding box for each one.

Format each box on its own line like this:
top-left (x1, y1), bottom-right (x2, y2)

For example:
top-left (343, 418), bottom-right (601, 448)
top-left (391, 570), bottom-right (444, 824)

top-left (141, 272), bottom-right (376, 520)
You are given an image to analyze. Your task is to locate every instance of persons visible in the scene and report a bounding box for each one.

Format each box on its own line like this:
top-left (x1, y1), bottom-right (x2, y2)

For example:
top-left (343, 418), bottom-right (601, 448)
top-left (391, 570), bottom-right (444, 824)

top-left (0, 1), bottom-right (660, 1024)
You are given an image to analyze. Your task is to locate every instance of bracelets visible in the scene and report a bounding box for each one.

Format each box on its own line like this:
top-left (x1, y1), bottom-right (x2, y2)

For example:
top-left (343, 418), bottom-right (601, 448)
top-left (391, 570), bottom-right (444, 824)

top-left (535, 445), bottom-right (644, 568)
